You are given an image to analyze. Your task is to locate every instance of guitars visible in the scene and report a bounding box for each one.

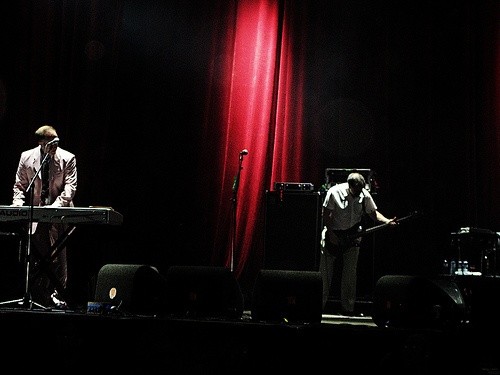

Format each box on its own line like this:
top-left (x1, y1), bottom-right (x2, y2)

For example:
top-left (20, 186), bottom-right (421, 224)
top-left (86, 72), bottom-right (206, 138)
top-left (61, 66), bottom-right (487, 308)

top-left (328, 207), bottom-right (416, 247)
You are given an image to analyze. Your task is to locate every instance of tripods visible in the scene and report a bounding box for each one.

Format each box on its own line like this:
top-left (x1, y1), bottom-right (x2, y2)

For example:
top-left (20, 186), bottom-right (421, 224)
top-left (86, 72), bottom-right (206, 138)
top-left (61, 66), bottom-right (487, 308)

top-left (0, 144), bottom-right (52, 310)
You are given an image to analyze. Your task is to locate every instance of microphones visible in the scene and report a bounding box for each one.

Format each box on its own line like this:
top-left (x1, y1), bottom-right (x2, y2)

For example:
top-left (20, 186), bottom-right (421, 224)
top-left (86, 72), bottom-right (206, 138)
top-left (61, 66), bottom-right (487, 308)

top-left (240, 150), bottom-right (248, 155)
top-left (47, 137), bottom-right (59, 144)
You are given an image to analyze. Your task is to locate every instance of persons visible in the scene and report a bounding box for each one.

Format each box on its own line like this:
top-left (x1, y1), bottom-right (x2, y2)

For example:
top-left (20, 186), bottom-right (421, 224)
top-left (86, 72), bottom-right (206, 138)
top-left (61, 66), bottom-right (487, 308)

top-left (11, 125), bottom-right (77, 310)
top-left (318, 172), bottom-right (400, 315)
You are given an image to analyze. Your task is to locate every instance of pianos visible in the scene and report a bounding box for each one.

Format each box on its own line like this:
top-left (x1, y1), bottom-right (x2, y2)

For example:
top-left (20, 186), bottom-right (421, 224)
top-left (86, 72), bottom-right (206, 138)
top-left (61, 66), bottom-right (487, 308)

top-left (0, 202), bottom-right (123, 228)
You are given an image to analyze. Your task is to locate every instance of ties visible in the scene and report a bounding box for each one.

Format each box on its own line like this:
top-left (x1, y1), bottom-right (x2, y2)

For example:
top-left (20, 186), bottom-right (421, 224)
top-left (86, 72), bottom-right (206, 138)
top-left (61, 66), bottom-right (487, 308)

top-left (41, 157), bottom-right (49, 207)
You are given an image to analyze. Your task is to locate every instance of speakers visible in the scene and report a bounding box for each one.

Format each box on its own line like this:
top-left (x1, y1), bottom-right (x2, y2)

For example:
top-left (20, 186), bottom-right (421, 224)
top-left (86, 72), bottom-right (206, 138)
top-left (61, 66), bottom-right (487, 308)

top-left (94, 263), bottom-right (324, 323)
top-left (371, 275), bottom-right (426, 326)
